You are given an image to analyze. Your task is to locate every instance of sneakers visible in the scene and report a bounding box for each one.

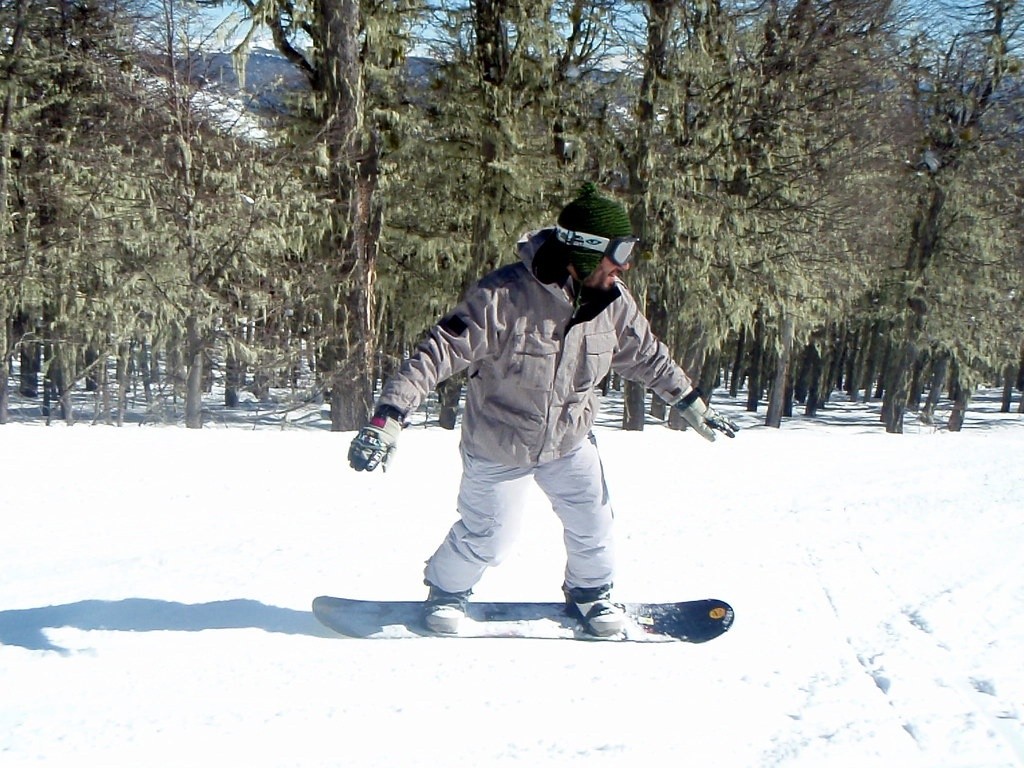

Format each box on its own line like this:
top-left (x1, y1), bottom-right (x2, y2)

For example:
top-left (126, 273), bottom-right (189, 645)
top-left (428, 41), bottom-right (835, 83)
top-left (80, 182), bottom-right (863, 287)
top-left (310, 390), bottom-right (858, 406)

top-left (560, 581), bottom-right (627, 637)
top-left (423, 578), bottom-right (474, 634)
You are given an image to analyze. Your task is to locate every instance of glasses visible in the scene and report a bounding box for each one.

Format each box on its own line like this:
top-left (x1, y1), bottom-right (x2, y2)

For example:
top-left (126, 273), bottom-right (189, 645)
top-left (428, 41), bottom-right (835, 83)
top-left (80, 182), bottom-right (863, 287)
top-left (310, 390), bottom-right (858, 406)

top-left (605, 237), bottom-right (641, 267)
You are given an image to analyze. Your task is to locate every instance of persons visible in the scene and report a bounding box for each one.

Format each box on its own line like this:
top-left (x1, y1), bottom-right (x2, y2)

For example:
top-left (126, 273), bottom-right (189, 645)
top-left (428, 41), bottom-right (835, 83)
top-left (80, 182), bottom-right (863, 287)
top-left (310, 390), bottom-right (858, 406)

top-left (348, 178), bottom-right (739, 636)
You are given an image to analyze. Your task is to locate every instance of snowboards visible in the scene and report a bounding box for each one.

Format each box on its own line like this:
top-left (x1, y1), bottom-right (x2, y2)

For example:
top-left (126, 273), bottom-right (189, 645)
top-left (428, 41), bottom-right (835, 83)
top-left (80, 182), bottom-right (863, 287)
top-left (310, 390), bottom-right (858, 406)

top-left (312, 595), bottom-right (735, 645)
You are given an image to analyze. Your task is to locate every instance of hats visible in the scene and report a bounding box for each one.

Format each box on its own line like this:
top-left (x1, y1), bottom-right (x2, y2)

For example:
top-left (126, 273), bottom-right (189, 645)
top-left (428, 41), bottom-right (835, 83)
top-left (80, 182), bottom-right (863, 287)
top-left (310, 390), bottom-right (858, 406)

top-left (558, 180), bottom-right (634, 281)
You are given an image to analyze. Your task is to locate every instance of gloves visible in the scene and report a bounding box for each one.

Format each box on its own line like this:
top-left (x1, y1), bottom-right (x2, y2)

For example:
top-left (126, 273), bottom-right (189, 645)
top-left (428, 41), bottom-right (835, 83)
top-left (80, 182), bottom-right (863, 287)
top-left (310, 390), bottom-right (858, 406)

top-left (668, 386), bottom-right (741, 443)
top-left (347, 405), bottom-right (411, 472)
